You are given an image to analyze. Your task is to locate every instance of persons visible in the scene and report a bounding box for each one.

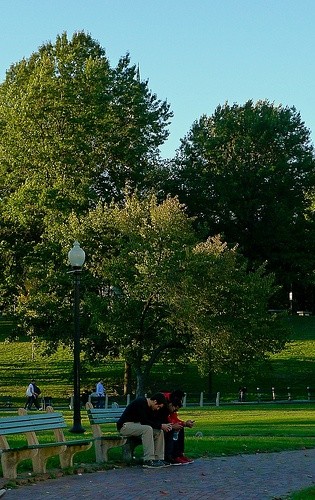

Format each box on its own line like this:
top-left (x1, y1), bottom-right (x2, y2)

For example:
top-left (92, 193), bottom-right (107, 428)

top-left (163, 391), bottom-right (193, 464)
top-left (117, 393), bottom-right (172, 468)
top-left (155, 399), bottom-right (181, 466)
top-left (96, 379), bottom-right (105, 408)
top-left (26, 380), bottom-right (42, 410)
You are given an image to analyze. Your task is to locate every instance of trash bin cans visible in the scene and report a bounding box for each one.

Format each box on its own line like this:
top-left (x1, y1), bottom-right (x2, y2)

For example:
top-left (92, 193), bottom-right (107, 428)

top-left (238, 388), bottom-right (247, 402)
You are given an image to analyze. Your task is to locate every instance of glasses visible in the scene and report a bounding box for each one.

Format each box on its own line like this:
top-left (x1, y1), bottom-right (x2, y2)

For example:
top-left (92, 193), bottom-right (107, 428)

top-left (175, 407), bottom-right (178, 411)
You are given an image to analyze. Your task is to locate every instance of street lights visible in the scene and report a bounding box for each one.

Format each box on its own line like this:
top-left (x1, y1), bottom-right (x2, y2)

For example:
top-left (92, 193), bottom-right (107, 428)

top-left (67, 239), bottom-right (86, 433)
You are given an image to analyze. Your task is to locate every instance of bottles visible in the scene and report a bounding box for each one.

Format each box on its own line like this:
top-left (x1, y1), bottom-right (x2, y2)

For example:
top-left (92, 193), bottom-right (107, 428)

top-left (173, 429), bottom-right (179, 441)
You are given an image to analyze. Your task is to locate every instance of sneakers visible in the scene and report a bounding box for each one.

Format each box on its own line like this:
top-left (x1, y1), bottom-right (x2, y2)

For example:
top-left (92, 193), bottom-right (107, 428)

top-left (161, 460), bottom-right (171, 466)
top-left (142, 460), bottom-right (161, 469)
top-left (165, 456), bottom-right (182, 466)
top-left (153, 460), bottom-right (167, 468)
top-left (183, 457), bottom-right (193, 463)
top-left (175, 457), bottom-right (189, 464)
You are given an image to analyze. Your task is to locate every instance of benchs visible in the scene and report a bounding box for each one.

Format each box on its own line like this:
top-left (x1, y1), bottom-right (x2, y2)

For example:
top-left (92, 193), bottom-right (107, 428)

top-left (184, 393), bottom-right (220, 408)
top-left (0, 406), bottom-right (94, 478)
top-left (89, 394), bottom-right (130, 407)
top-left (86, 402), bottom-right (143, 461)
